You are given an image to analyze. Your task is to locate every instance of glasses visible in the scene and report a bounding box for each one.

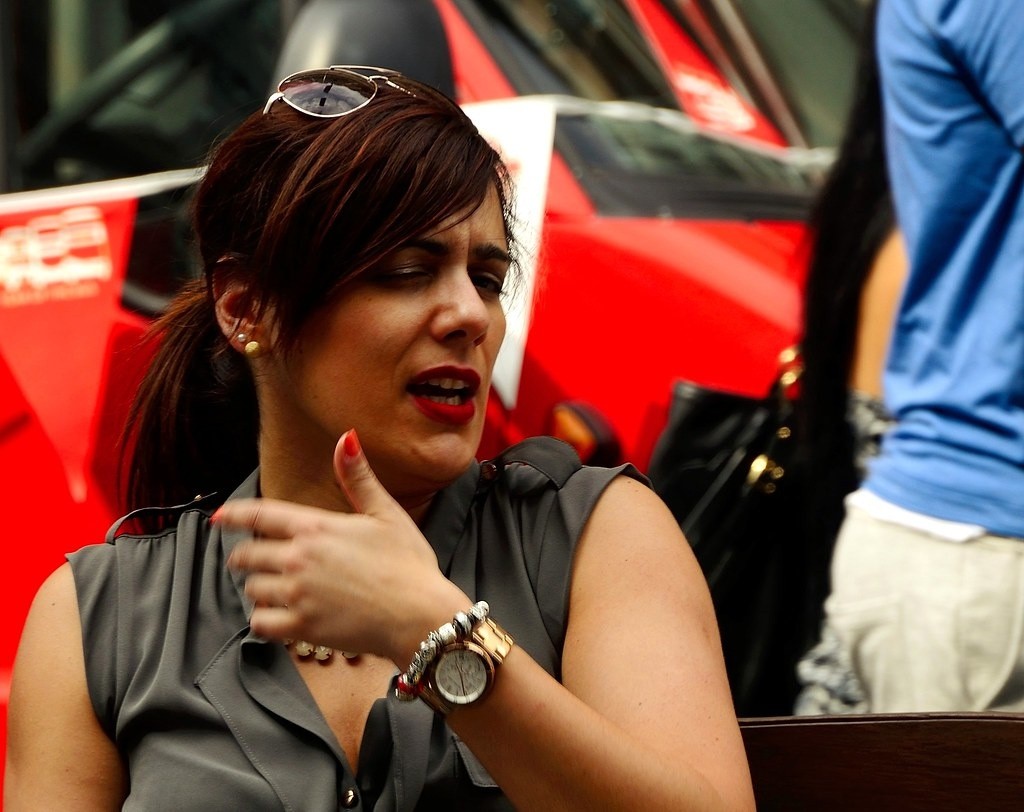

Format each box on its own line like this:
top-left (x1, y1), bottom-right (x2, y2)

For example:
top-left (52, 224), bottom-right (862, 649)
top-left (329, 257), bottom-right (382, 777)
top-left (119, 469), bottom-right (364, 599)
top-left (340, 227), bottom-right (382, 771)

top-left (262, 65), bottom-right (471, 126)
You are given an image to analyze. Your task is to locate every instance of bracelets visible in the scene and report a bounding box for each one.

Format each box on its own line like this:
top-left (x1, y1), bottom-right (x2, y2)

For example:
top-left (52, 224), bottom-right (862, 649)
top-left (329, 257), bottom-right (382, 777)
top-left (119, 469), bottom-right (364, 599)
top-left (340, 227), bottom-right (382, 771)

top-left (395, 601), bottom-right (490, 702)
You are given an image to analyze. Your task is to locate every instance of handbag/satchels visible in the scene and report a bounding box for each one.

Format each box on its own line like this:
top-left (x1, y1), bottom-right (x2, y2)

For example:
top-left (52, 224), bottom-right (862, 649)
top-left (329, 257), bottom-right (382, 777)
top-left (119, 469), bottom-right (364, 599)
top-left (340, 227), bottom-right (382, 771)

top-left (645, 380), bottom-right (858, 716)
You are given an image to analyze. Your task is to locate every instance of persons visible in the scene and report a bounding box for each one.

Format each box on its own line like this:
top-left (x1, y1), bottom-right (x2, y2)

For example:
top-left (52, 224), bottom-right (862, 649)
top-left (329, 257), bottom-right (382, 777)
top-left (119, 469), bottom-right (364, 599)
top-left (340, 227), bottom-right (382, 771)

top-left (795, 0), bottom-right (1024, 716)
top-left (3, 65), bottom-right (757, 812)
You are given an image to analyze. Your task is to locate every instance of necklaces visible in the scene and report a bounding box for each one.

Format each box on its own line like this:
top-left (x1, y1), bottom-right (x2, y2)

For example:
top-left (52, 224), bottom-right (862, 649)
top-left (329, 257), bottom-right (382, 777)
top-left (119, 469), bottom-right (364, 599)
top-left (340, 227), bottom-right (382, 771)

top-left (282, 638), bottom-right (359, 660)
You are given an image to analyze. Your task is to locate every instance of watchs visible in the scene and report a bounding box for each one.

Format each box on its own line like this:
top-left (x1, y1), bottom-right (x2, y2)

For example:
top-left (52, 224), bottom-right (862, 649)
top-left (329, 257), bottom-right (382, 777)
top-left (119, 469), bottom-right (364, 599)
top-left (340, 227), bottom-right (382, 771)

top-left (419, 616), bottom-right (515, 718)
